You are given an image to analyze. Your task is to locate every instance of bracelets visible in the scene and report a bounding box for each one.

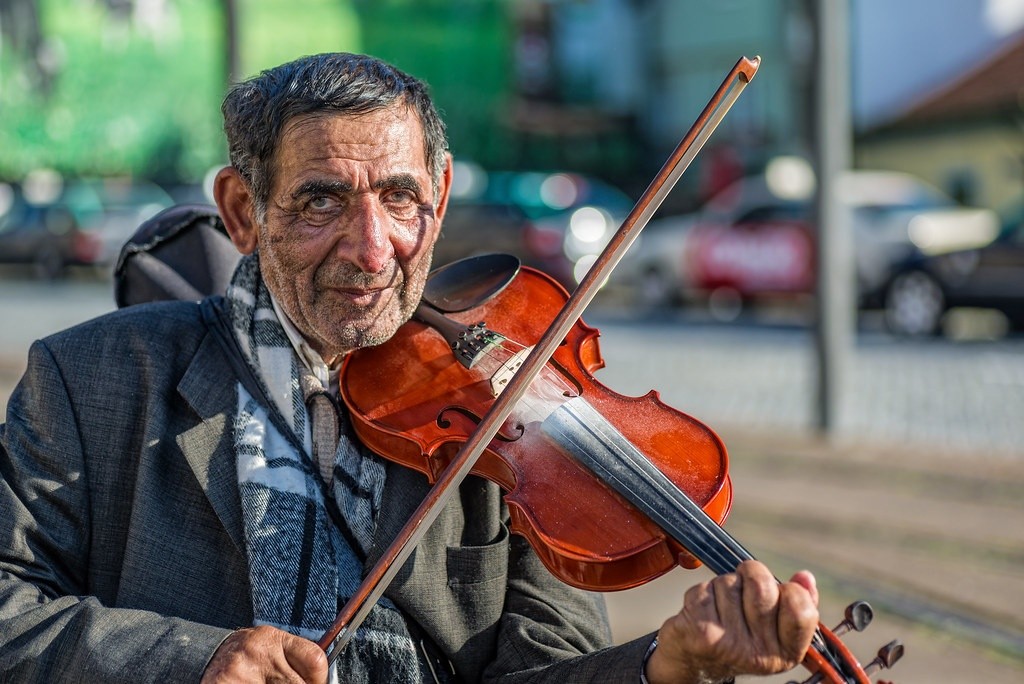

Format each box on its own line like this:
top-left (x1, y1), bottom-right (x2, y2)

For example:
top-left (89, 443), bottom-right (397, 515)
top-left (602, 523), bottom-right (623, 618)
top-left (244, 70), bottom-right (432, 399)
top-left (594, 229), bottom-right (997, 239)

top-left (639, 634), bottom-right (660, 684)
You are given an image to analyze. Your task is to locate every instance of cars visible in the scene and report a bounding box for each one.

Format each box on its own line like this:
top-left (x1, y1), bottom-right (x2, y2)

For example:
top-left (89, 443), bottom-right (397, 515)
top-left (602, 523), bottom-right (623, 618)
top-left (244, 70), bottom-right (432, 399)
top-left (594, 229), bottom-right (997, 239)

top-left (628, 166), bottom-right (974, 324)
top-left (0, 174), bottom-right (198, 294)
top-left (420, 165), bottom-right (648, 307)
top-left (856, 187), bottom-right (1023, 341)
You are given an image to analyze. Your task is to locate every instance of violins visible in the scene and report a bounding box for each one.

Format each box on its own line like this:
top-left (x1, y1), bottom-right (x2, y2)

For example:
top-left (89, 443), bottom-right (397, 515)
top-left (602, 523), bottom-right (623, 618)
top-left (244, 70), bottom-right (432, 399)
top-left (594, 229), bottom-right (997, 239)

top-left (336, 261), bottom-right (910, 684)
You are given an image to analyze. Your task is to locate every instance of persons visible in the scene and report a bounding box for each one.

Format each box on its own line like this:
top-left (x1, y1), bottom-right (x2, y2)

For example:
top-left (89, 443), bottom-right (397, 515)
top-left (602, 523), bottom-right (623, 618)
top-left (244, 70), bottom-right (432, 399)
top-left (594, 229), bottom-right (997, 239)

top-left (0, 52), bottom-right (818, 684)
